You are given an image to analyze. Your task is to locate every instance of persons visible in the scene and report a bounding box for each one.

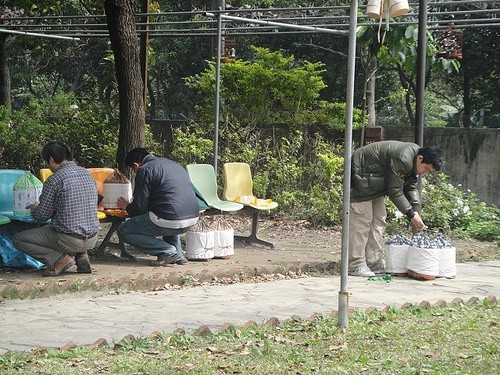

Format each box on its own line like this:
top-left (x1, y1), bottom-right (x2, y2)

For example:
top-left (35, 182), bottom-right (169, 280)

top-left (117, 146), bottom-right (197, 267)
top-left (344, 140), bottom-right (442, 278)
top-left (11, 140), bottom-right (100, 277)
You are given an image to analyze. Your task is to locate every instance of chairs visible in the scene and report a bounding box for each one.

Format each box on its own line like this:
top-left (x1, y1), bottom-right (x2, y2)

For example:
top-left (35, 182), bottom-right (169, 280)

top-left (0, 162), bottom-right (278, 260)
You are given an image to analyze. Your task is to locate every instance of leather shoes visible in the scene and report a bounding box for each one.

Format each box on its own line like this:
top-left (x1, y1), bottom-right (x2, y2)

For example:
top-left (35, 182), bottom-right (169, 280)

top-left (75, 253), bottom-right (91, 274)
top-left (44, 253), bottom-right (76, 277)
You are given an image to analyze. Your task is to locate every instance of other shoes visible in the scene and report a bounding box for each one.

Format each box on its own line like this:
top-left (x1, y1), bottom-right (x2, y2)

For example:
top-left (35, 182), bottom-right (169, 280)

top-left (368, 260), bottom-right (388, 274)
top-left (151, 251), bottom-right (179, 266)
top-left (349, 263), bottom-right (375, 277)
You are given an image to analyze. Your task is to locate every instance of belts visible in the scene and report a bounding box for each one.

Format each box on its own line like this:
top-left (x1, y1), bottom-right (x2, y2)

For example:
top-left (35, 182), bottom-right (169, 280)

top-left (66, 232), bottom-right (98, 240)
